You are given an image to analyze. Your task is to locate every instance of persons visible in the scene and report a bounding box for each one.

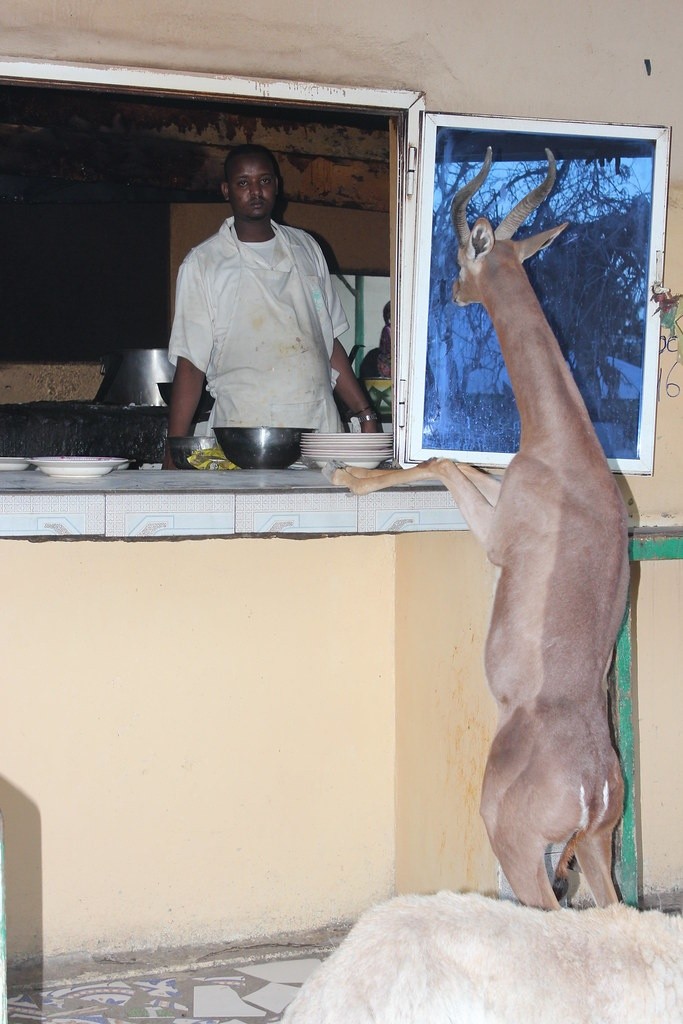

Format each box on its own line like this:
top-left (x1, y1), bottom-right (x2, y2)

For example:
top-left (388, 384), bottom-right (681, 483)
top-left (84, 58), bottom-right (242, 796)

top-left (161, 145), bottom-right (383, 473)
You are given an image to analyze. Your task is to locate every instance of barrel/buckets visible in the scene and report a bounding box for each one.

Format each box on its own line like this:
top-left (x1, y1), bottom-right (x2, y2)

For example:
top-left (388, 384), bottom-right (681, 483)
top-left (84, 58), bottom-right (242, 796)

top-left (121, 348), bottom-right (177, 406)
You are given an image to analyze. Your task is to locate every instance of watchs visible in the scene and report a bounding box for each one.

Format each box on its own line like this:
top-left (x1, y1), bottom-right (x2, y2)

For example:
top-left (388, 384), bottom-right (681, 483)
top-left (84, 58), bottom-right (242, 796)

top-left (358, 412), bottom-right (377, 424)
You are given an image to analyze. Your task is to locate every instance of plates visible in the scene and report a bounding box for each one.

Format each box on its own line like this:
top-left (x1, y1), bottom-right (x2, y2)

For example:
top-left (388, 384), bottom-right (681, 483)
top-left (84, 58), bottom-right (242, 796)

top-left (33, 456), bottom-right (136, 471)
top-left (27, 458), bottom-right (128, 478)
top-left (0, 457), bottom-right (30, 471)
top-left (300, 432), bottom-right (394, 469)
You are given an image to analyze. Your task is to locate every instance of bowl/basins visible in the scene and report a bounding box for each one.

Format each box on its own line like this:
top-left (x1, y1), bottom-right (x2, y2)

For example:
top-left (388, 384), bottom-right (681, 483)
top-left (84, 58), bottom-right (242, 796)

top-left (158, 383), bottom-right (209, 408)
top-left (211, 427), bottom-right (318, 470)
top-left (167, 437), bottom-right (216, 470)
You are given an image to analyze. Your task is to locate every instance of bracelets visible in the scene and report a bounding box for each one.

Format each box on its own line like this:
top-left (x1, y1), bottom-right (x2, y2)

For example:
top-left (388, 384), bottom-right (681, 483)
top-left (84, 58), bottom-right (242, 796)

top-left (354, 401), bottom-right (372, 415)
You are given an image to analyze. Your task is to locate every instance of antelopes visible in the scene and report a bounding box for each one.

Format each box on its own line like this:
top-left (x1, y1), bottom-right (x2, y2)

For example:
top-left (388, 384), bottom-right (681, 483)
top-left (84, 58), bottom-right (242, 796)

top-left (321, 147), bottom-right (633, 911)
